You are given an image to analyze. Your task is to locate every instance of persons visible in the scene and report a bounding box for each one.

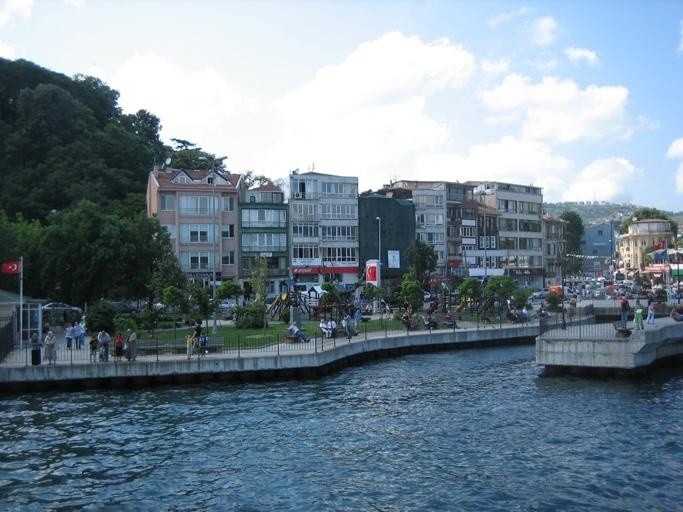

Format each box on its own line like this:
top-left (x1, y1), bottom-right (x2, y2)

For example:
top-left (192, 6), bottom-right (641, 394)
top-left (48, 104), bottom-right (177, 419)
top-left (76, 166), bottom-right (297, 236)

top-left (402, 301), bottom-right (459, 329)
top-left (31, 319), bottom-right (138, 365)
top-left (185, 318), bottom-right (206, 359)
top-left (569, 295), bottom-right (577, 319)
top-left (507, 302), bottom-right (552, 324)
top-left (287, 310), bottom-right (362, 342)
top-left (619, 293), bottom-right (656, 331)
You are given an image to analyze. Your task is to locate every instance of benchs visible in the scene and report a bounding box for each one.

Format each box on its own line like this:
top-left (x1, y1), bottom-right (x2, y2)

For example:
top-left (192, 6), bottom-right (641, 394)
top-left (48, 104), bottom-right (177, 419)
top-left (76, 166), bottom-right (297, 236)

top-left (285, 329), bottom-right (301, 344)
top-left (136, 336), bottom-right (224, 355)
top-left (319, 324), bottom-right (346, 338)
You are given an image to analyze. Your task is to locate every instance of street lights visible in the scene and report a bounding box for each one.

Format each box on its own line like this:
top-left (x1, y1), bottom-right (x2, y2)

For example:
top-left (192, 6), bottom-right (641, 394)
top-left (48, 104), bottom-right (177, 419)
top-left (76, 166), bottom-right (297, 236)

top-left (375, 215), bottom-right (382, 312)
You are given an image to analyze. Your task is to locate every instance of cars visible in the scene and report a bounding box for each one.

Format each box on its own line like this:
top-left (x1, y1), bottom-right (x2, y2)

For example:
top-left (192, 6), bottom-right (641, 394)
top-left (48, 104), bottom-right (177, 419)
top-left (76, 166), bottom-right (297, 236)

top-left (354, 296), bottom-right (369, 306)
top-left (422, 291), bottom-right (430, 302)
top-left (36, 296), bottom-right (266, 319)
top-left (373, 297), bottom-right (388, 307)
top-left (499, 277), bottom-right (682, 305)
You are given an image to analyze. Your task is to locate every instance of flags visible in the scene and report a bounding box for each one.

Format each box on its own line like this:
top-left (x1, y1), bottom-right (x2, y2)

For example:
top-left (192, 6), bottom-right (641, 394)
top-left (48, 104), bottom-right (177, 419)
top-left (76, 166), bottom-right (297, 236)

top-left (2, 261), bottom-right (20, 274)
